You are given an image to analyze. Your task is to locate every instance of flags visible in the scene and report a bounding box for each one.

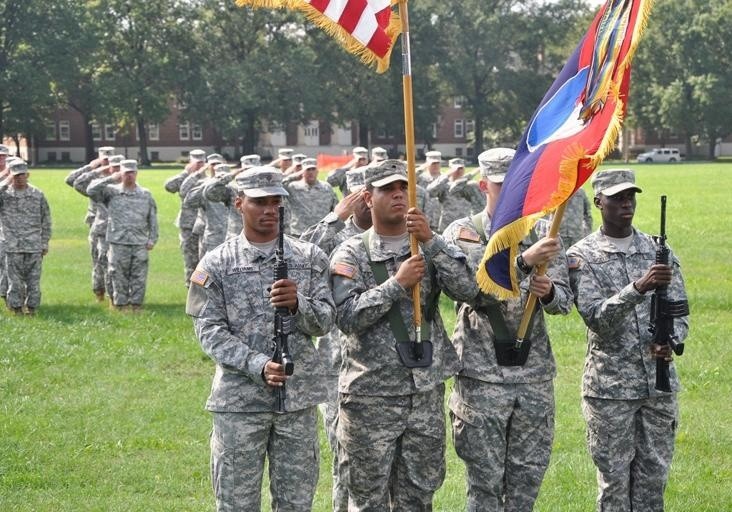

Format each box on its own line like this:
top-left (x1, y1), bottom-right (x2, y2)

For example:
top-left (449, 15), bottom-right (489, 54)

top-left (475, 1), bottom-right (653, 303)
top-left (234, 0), bottom-right (403, 74)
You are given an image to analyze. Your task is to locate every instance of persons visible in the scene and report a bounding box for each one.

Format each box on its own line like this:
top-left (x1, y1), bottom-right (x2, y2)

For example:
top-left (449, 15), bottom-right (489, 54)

top-left (64, 147), bottom-right (159, 309)
top-left (566, 169), bottom-right (689, 512)
top-left (166, 150), bottom-right (262, 288)
top-left (550, 188), bottom-right (592, 249)
top-left (324, 158), bottom-right (481, 511)
top-left (440, 148), bottom-right (575, 512)
top-left (185, 165), bottom-right (336, 512)
top-left (269, 147), bottom-right (486, 241)
top-left (1, 147), bottom-right (51, 315)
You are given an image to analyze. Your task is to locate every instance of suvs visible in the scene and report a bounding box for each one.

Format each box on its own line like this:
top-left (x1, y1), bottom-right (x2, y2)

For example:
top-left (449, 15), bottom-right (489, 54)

top-left (604, 147), bottom-right (682, 163)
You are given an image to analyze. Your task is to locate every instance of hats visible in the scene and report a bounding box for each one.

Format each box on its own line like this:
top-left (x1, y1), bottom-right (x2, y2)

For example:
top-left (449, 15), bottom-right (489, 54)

top-left (448, 157), bottom-right (465, 168)
top-left (372, 147), bottom-right (389, 160)
top-left (352, 147), bottom-right (368, 159)
top-left (591, 169), bottom-right (643, 197)
top-left (346, 166), bottom-right (369, 192)
top-left (425, 151), bottom-right (442, 163)
top-left (363, 158), bottom-right (408, 191)
top-left (235, 166), bottom-right (290, 199)
top-left (477, 147), bottom-right (516, 185)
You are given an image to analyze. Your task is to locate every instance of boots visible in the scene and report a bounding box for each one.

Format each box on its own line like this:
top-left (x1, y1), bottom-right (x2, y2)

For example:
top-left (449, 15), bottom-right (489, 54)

top-left (4, 295), bottom-right (36, 316)
top-left (96, 290), bottom-right (141, 313)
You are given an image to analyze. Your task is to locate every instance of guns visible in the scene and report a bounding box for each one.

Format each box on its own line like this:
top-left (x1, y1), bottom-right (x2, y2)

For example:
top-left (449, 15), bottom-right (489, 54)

top-left (648, 195), bottom-right (688, 391)
top-left (273, 207), bottom-right (294, 414)
